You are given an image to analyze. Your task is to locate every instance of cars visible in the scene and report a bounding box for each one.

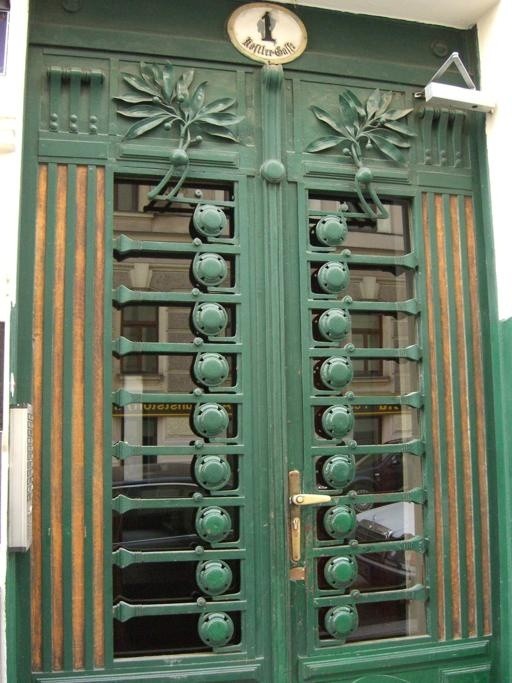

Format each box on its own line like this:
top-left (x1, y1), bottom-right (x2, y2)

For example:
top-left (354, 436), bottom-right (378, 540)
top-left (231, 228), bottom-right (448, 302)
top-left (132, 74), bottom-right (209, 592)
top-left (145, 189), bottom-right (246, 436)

top-left (111, 464), bottom-right (247, 651)
top-left (349, 500), bottom-right (408, 591)
top-left (321, 437), bottom-right (405, 515)
